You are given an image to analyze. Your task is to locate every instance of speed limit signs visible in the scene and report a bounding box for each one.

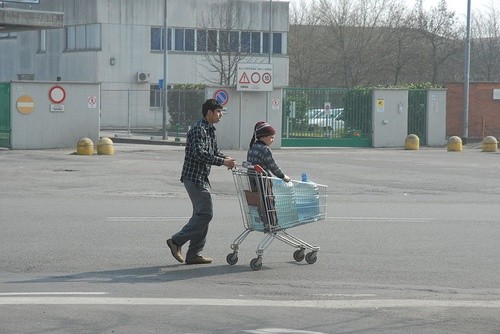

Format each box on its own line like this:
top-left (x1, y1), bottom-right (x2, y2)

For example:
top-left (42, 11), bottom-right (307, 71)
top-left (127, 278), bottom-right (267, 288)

top-left (262, 73), bottom-right (271, 84)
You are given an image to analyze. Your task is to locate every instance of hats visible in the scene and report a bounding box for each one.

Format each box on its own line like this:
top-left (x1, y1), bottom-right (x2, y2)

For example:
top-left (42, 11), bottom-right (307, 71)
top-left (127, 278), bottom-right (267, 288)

top-left (250, 121), bottom-right (276, 149)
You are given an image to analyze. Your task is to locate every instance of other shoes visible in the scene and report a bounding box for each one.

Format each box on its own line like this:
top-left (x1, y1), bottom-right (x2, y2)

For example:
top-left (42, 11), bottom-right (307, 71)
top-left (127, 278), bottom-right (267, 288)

top-left (185, 256), bottom-right (212, 264)
top-left (263, 226), bottom-right (282, 231)
top-left (166, 238), bottom-right (184, 263)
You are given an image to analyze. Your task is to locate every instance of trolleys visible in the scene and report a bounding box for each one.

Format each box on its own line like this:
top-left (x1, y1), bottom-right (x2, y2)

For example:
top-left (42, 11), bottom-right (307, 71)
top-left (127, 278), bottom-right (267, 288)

top-left (226, 161), bottom-right (328, 271)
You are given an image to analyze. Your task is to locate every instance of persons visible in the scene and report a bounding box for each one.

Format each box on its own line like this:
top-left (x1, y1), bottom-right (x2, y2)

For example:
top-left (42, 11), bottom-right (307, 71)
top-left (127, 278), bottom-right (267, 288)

top-left (167, 99), bottom-right (236, 264)
top-left (247, 122), bottom-right (290, 227)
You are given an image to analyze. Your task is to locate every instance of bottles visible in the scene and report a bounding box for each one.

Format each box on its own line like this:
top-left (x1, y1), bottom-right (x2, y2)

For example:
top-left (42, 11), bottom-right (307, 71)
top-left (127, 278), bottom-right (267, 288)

top-left (294, 172), bottom-right (320, 222)
top-left (272, 178), bottom-right (297, 227)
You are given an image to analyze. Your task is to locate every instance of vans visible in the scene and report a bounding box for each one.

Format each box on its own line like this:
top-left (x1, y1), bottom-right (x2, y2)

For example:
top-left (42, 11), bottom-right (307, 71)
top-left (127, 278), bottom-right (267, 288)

top-left (296, 109), bottom-right (344, 134)
